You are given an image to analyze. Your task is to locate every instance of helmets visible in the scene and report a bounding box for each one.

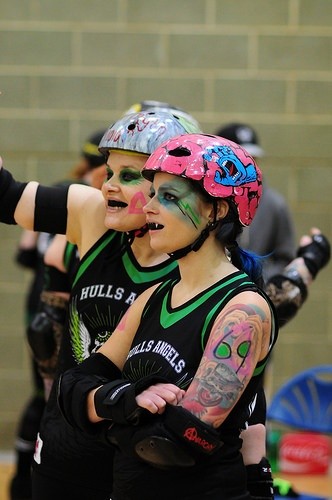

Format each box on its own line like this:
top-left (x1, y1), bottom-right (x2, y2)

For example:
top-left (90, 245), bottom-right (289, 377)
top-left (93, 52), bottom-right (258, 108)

top-left (97, 107), bottom-right (201, 157)
top-left (142, 132), bottom-right (263, 227)
top-left (84, 132), bottom-right (111, 158)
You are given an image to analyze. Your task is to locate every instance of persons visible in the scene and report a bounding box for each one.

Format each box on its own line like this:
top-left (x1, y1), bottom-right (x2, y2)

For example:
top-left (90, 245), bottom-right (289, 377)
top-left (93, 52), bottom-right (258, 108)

top-left (259, 225), bottom-right (332, 332)
top-left (214, 122), bottom-right (296, 288)
top-left (56, 131), bottom-right (276, 500)
top-left (0, 100), bottom-right (276, 500)
top-left (12, 123), bottom-right (105, 500)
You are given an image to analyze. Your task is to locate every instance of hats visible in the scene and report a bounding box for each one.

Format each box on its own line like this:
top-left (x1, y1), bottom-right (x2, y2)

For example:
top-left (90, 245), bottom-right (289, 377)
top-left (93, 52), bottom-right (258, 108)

top-left (218, 126), bottom-right (263, 158)
